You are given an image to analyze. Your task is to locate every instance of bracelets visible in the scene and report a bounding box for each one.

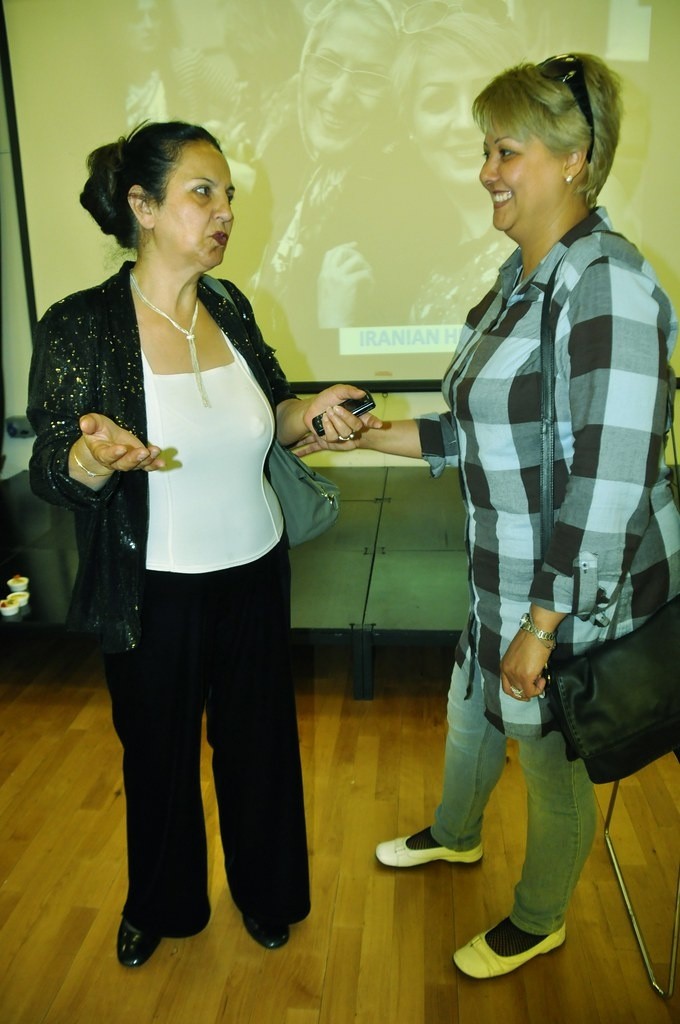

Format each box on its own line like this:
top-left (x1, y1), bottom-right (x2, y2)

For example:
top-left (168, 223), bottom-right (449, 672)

top-left (529, 612), bottom-right (556, 649)
top-left (72, 444), bottom-right (113, 477)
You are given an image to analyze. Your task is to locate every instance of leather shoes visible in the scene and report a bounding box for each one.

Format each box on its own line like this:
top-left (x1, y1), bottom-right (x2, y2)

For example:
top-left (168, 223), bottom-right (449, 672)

top-left (116, 917), bottom-right (167, 967)
top-left (239, 912), bottom-right (290, 949)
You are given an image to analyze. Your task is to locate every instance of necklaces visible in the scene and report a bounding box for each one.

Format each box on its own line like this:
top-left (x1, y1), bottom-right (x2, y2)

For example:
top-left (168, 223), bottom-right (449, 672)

top-left (129, 270), bottom-right (211, 409)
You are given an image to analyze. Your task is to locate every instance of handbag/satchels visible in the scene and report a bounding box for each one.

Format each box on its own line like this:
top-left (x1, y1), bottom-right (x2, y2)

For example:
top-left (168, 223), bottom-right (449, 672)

top-left (542, 594), bottom-right (680, 785)
top-left (198, 272), bottom-right (339, 548)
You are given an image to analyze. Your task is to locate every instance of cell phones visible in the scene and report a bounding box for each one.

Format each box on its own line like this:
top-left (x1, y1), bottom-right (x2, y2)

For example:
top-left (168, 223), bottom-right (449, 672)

top-left (312, 391), bottom-right (375, 436)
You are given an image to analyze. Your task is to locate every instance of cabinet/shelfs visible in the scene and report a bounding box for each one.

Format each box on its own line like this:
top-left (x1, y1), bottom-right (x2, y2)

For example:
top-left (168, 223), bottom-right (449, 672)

top-left (290, 393), bottom-right (470, 700)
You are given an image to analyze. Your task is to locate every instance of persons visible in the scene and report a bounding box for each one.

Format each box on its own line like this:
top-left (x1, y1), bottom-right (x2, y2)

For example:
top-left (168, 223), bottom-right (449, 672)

top-left (25, 120), bottom-right (383, 967)
top-left (288, 52), bottom-right (679, 979)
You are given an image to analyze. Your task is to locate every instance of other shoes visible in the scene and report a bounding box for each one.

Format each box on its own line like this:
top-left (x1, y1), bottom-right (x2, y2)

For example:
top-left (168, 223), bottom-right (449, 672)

top-left (374, 825), bottom-right (484, 868)
top-left (451, 914), bottom-right (568, 979)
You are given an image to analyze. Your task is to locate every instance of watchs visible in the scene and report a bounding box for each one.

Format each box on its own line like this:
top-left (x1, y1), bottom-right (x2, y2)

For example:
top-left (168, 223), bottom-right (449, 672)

top-left (519, 613), bottom-right (557, 641)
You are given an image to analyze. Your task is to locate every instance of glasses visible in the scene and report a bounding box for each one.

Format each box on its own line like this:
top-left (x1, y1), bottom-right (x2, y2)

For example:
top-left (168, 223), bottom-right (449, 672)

top-left (536, 53), bottom-right (596, 164)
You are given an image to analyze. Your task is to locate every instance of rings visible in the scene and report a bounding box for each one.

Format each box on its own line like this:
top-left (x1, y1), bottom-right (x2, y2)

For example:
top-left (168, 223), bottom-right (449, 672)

top-left (338, 434), bottom-right (354, 441)
top-left (510, 685), bottom-right (524, 699)
